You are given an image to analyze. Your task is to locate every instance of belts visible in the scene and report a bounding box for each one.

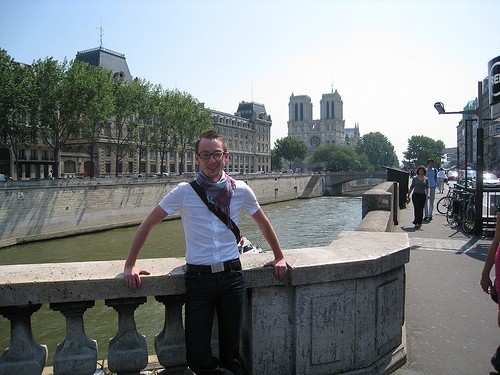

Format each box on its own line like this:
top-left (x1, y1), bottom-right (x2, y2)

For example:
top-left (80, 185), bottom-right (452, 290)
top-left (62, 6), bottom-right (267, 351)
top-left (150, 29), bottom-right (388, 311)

top-left (186, 259), bottom-right (242, 273)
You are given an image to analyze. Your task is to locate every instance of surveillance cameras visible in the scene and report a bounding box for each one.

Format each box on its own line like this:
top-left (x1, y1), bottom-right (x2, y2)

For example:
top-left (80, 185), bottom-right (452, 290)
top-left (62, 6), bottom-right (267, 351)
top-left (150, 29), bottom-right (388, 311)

top-left (434, 102), bottom-right (445, 114)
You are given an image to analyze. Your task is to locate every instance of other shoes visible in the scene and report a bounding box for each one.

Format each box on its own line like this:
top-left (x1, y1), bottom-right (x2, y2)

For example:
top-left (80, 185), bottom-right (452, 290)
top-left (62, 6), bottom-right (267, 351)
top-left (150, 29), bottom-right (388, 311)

top-left (424, 217), bottom-right (432, 221)
top-left (414, 224), bottom-right (422, 230)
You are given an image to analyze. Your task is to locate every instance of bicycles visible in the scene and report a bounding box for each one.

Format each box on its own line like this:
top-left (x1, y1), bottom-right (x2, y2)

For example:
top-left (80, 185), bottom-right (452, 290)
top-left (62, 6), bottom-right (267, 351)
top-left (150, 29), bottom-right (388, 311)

top-left (437, 184), bottom-right (477, 233)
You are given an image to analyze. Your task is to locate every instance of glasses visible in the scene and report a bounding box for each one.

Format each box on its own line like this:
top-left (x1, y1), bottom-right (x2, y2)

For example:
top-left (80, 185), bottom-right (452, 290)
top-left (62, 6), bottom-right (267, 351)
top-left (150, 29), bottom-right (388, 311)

top-left (198, 151), bottom-right (224, 159)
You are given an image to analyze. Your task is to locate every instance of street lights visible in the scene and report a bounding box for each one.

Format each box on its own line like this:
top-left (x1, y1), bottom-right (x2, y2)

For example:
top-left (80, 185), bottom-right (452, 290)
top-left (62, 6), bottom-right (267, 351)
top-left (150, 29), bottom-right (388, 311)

top-left (433, 81), bottom-right (482, 236)
top-left (464, 116), bottom-right (500, 185)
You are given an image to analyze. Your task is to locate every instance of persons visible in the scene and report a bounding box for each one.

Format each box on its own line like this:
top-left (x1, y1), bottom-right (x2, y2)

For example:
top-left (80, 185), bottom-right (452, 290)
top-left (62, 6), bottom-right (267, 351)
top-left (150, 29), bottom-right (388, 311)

top-left (437, 167), bottom-right (446, 194)
top-left (423, 159), bottom-right (439, 221)
top-left (123, 130), bottom-right (288, 375)
top-left (480, 210), bottom-right (500, 328)
top-left (410, 170), bottom-right (414, 178)
top-left (406, 165), bottom-right (431, 228)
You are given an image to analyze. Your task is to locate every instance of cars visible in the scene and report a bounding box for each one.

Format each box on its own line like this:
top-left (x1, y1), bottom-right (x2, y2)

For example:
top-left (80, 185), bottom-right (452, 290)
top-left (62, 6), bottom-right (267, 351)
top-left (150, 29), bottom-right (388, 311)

top-left (447, 171), bottom-right (458, 180)
top-left (472, 172), bottom-right (500, 192)
top-left (458, 170), bottom-right (476, 184)
